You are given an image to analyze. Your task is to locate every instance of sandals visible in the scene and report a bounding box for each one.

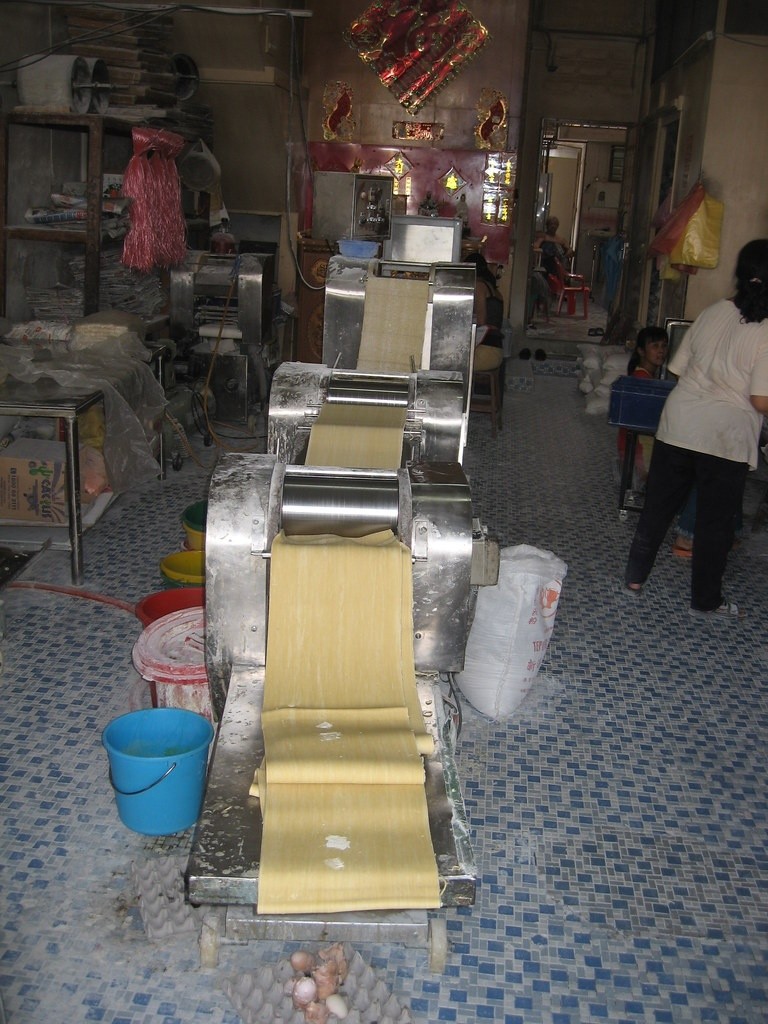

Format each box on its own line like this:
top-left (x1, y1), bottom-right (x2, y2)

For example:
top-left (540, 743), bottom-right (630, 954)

top-left (623, 580), bottom-right (643, 597)
top-left (688, 600), bottom-right (747, 619)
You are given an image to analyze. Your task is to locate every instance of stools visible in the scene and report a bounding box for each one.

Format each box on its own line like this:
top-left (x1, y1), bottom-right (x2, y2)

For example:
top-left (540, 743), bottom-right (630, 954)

top-left (470, 345), bottom-right (505, 433)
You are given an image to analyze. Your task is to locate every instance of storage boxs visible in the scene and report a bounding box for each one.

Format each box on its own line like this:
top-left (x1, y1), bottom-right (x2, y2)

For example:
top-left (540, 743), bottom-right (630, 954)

top-left (606, 375), bottom-right (678, 429)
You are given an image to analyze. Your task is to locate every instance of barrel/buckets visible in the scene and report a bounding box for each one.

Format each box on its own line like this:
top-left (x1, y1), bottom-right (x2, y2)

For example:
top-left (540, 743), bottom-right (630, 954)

top-left (100, 706), bottom-right (214, 835)
top-left (181, 500), bottom-right (208, 550)
top-left (132, 605), bottom-right (214, 725)
top-left (160, 550), bottom-right (206, 588)
top-left (134, 587), bottom-right (205, 629)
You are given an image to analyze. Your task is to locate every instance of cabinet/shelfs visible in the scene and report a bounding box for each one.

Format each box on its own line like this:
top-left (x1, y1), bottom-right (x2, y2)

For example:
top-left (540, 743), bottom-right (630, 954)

top-left (617, 430), bottom-right (657, 521)
top-left (0, 105), bottom-right (231, 343)
top-left (0, 344), bottom-right (175, 587)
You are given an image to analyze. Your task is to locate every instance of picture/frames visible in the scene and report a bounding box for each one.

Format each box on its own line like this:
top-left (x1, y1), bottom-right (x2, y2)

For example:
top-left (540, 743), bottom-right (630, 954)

top-left (608, 145), bottom-right (625, 182)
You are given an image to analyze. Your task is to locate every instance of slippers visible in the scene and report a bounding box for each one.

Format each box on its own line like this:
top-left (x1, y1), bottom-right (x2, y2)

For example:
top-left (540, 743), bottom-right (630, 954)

top-left (672, 543), bottom-right (691, 557)
top-left (588, 327), bottom-right (606, 336)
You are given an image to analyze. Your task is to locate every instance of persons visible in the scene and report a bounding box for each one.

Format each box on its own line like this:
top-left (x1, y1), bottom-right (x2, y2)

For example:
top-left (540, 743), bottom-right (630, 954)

top-left (618, 238), bottom-right (768, 621)
top-left (465, 252), bottom-right (504, 333)
top-left (534, 215), bottom-right (575, 298)
top-left (418, 191), bottom-right (440, 218)
top-left (454, 194), bottom-right (469, 223)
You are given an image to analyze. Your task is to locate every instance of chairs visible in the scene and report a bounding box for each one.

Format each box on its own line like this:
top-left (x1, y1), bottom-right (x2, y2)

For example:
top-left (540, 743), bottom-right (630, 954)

top-left (554, 257), bottom-right (591, 319)
top-left (534, 247), bottom-right (574, 273)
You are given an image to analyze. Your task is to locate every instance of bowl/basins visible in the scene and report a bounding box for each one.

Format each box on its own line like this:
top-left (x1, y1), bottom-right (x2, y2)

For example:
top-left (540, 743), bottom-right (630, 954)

top-left (336, 240), bottom-right (382, 258)
